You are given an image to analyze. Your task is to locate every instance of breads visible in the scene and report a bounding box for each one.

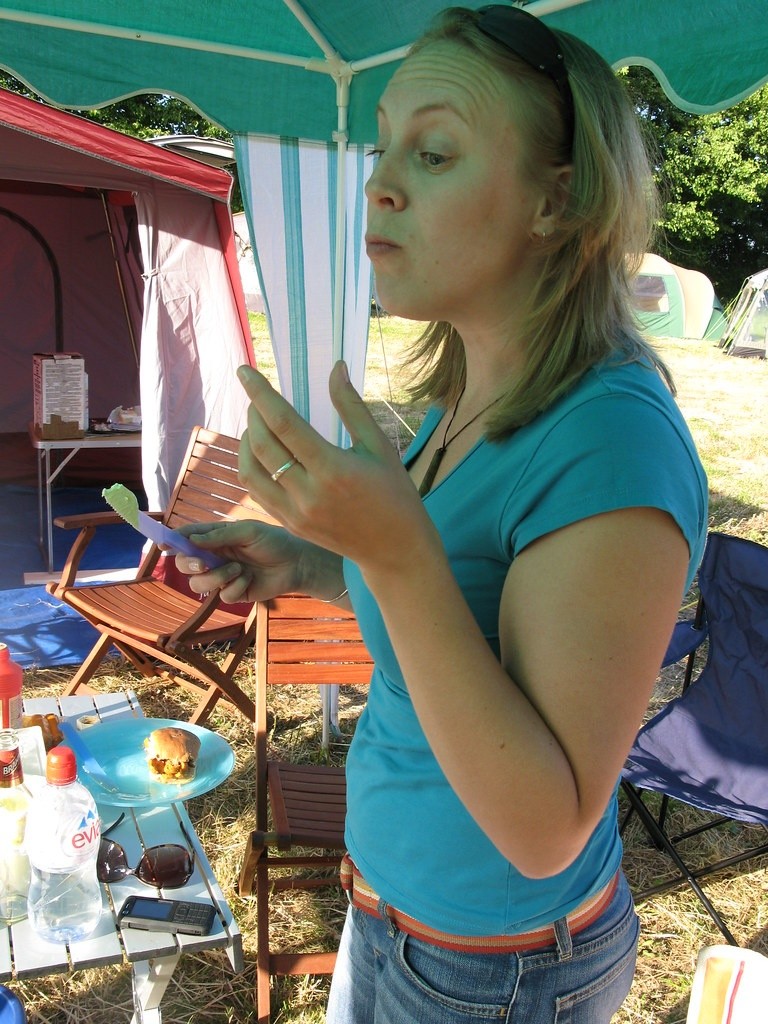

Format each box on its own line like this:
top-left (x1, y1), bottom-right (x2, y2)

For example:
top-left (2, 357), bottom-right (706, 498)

top-left (23, 713), bottom-right (63, 750)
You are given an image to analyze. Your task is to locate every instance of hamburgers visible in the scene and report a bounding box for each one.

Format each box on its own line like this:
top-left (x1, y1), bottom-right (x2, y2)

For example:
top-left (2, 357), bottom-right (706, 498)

top-left (143, 727), bottom-right (201, 784)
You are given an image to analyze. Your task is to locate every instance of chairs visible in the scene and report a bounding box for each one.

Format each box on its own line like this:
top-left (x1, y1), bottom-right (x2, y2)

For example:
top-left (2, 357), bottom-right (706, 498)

top-left (234, 591), bottom-right (376, 1024)
top-left (616, 532), bottom-right (768, 948)
top-left (46, 425), bottom-right (284, 727)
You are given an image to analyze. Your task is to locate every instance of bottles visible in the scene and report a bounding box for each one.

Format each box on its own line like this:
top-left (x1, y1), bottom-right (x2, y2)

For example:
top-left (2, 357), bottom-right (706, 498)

top-left (25, 746), bottom-right (102, 944)
top-left (0, 642), bottom-right (22, 729)
top-left (0, 730), bottom-right (27, 924)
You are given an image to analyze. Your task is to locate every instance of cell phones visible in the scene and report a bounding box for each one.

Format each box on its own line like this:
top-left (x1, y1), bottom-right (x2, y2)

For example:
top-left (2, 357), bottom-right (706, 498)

top-left (116, 895), bottom-right (216, 936)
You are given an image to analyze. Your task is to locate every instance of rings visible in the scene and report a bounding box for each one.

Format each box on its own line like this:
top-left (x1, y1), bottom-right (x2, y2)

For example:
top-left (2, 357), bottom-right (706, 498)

top-left (271, 460), bottom-right (296, 480)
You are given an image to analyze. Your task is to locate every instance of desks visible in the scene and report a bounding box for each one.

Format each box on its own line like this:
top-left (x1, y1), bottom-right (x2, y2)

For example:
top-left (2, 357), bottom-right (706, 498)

top-left (0, 689), bottom-right (243, 1024)
top-left (24, 418), bottom-right (141, 583)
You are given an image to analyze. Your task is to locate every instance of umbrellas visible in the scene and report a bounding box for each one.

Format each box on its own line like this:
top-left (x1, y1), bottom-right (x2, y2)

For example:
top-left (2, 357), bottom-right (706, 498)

top-left (1, 0), bottom-right (768, 755)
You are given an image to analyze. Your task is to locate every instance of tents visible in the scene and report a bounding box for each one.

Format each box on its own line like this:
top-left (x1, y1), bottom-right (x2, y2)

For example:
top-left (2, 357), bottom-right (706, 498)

top-left (625, 250), bottom-right (767, 359)
top-left (1, 86), bottom-right (257, 591)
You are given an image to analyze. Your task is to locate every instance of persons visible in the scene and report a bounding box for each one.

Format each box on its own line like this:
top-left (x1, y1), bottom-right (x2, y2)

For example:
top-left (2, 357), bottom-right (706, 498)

top-left (161, 5), bottom-right (711, 1024)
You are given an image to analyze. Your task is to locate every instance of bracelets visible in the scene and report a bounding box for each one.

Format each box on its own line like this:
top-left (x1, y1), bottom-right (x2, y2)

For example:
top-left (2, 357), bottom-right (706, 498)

top-left (320, 588), bottom-right (348, 604)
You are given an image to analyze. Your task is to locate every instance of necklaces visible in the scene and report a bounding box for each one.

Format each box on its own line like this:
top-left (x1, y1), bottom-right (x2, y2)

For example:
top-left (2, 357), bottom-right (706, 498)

top-left (416, 382), bottom-right (509, 497)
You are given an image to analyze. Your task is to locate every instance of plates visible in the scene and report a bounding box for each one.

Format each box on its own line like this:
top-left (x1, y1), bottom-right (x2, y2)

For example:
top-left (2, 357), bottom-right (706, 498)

top-left (52, 717), bottom-right (234, 808)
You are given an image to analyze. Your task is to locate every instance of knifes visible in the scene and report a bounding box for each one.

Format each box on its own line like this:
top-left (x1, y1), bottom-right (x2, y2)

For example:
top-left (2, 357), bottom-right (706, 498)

top-left (102, 488), bottom-right (225, 569)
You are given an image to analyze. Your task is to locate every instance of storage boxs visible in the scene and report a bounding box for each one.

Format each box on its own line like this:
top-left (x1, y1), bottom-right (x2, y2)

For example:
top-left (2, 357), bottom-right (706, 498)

top-left (33, 352), bottom-right (88, 439)
